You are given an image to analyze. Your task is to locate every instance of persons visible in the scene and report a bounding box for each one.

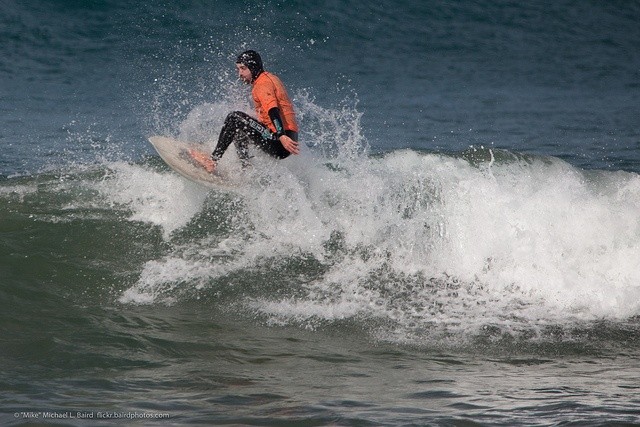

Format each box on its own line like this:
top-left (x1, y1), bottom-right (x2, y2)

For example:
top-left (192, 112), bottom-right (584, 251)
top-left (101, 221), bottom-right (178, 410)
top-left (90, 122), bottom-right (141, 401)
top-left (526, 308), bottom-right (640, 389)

top-left (184, 48), bottom-right (302, 176)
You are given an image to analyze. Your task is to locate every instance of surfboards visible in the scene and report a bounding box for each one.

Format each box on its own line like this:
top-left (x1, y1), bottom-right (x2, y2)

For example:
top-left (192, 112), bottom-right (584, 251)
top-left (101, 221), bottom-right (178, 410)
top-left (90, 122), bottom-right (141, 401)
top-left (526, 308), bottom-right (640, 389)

top-left (149, 135), bottom-right (244, 192)
